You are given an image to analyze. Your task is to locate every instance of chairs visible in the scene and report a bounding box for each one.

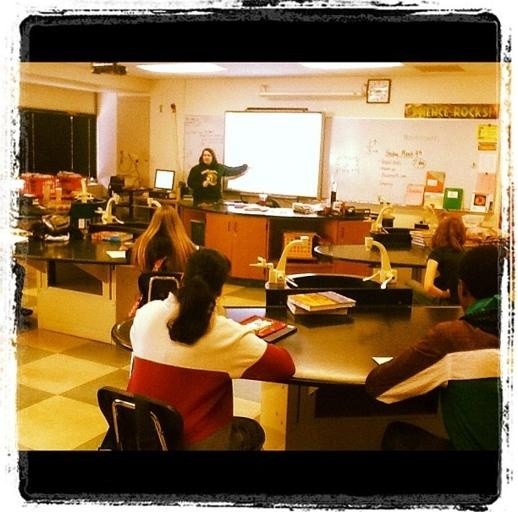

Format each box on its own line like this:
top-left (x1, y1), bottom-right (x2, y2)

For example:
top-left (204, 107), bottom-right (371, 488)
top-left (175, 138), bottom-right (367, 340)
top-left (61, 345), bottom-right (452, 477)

top-left (96, 387), bottom-right (183, 450)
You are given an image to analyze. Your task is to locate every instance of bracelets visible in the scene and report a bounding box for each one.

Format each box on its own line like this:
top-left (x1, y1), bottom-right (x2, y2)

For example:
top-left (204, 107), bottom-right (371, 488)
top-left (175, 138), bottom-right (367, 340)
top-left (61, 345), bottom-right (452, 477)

top-left (439, 289), bottom-right (447, 301)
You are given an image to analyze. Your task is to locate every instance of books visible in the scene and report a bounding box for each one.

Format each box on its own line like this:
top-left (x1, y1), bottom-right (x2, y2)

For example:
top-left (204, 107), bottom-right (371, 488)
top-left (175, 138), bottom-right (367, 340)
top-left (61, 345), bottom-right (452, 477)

top-left (288, 291), bottom-right (358, 311)
top-left (408, 231), bottom-right (439, 248)
top-left (285, 302), bottom-right (349, 316)
top-left (238, 314), bottom-right (287, 338)
top-left (90, 229), bottom-right (134, 242)
top-left (405, 170), bottom-right (491, 213)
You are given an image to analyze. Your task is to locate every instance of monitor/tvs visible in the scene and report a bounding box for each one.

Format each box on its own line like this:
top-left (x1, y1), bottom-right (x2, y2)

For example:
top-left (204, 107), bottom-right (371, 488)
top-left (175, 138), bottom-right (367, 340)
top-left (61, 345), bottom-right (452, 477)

top-left (154, 169), bottom-right (176, 199)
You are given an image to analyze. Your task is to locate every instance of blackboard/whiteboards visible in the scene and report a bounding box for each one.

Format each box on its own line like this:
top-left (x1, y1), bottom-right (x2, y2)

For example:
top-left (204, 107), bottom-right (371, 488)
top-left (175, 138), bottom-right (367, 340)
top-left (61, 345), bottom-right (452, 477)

top-left (328, 116), bottom-right (501, 215)
top-left (183, 116), bottom-right (329, 200)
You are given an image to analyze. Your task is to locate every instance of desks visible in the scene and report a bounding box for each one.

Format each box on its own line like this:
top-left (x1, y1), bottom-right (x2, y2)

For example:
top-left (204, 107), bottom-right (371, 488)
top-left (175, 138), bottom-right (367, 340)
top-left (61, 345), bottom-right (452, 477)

top-left (14, 231), bottom-right (137, 348)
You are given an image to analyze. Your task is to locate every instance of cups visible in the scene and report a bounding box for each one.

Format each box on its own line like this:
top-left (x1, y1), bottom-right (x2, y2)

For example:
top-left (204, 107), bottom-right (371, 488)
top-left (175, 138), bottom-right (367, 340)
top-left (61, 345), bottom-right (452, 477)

top-left (364, 236), bottom-right (373, 252)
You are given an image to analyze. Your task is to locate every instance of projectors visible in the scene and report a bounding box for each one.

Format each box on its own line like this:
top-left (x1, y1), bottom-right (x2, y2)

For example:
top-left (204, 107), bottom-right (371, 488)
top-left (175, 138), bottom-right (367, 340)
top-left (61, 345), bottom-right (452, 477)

top-left (91, 65), bottom-right (126, 75)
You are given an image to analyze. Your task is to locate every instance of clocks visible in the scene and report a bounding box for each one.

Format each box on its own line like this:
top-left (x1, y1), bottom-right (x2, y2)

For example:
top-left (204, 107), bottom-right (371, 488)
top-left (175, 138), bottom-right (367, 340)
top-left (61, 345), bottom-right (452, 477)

top-left (366, 78), bottom-right (391, 104)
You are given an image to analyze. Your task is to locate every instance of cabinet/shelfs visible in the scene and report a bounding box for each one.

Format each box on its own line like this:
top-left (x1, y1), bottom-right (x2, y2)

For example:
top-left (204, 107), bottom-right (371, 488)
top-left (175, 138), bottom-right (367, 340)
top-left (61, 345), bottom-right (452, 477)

top-left (205, 212), bottom-right (269, 284)
top-left (320, 220), bottom-right (373, 279)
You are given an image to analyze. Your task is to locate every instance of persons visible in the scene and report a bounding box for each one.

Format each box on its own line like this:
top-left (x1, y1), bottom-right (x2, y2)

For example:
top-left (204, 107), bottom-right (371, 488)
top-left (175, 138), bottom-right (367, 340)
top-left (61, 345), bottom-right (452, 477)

top-left (127, 248), bottom-right (297, 450)
top-left (132, 204), bottom-right (197, 272)
top-left (186, 147), bottom-right (249, 203)
top-left (423, 217), bottom-right (469, 303)
top-left (363, 242), bottom-right (504, 451)
